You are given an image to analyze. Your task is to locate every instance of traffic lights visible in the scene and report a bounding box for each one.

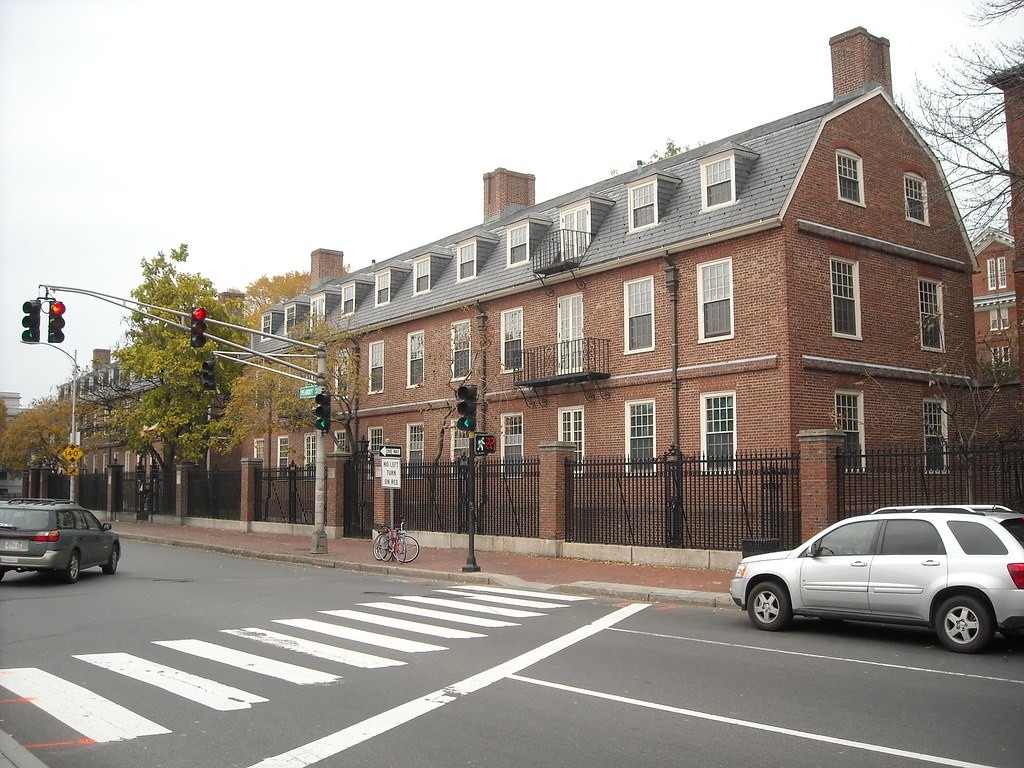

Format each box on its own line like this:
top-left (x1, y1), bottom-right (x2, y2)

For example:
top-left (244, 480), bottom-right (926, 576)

top-left (314, 392), bottom-right (332, 432)
top-left (456, 385), bottom-right (478, 430)
top-left (201, 359), bottom-right (216, 391)
top-left (46, 301), bottom-right (67, 344)
top-left (474, 434), bottom-right (496, 455)
top-left (22, 299), bottom-right (42, 342)
top-left (189, 306), bottom-right (208, 349)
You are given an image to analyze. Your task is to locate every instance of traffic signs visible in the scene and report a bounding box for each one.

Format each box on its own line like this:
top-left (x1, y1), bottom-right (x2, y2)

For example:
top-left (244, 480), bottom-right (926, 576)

top-left (378, 444), bottom-right (402, 457)
top-left (61, 443), bottom-right (85, 466)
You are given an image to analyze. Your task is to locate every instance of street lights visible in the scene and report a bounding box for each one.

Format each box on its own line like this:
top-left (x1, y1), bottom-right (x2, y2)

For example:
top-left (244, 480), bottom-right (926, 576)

top-left (19, 340), bottom-right (80, 505)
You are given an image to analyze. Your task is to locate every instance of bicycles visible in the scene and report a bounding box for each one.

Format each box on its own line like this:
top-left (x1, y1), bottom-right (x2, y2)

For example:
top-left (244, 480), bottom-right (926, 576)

top-left (372, 520), bottom-right (420, 565)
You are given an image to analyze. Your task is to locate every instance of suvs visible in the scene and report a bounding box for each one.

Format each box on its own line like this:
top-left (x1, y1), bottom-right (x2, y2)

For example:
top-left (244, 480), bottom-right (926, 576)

top-left (0, 498), bottom-right (122, 585)
top-left (728, 503), bottom-right (1024, 656)
